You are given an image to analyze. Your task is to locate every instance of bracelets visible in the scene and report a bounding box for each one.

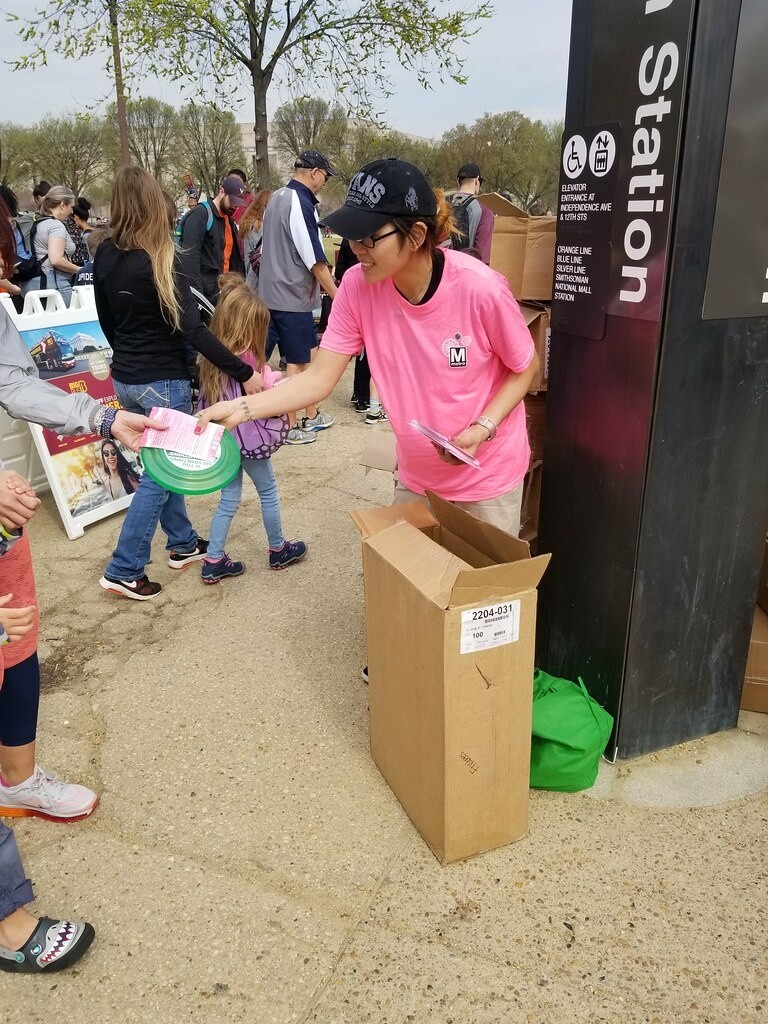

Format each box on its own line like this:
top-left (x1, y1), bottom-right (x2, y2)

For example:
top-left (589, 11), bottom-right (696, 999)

top-left (471, 416), bottom-right (497, 442)
top-left (95, 406), bottom-right (118, 438)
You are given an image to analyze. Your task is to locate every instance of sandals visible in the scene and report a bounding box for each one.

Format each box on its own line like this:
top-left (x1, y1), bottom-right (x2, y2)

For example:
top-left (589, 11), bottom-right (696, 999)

top-left (0, 915), bottom-right (95, 974)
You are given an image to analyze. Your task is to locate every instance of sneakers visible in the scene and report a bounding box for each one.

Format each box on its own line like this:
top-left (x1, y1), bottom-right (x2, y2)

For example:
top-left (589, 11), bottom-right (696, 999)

top-left (355, 400), bottom-right (370, 412)
top-left (286, 421), bottom-right (318, 444)
top-left (200, 551), bottom-right (245, 584)
top-left (168, 536), bottom-right (210, 570)
top-left (268, 539), bottom-right (308, 571)
top-left (350, 393), bottom-right (359, 403)
top-left (0, 763), bottom-right (100, 824)
top-left (365, 411), bottom-right (389, 424)
top-left (301, 411), bottom-right (334, 432)
top-left (98, 574), bottom-right (162, 601)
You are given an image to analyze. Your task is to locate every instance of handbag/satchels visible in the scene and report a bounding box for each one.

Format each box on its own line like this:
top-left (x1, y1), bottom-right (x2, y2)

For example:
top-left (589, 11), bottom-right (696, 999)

top-left (529, 667), bottom-right (615, 795)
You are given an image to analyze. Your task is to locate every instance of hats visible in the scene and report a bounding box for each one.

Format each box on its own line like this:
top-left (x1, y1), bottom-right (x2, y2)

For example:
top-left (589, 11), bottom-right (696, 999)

top-left (317, 158), bottom-right (438, 241)
top-left (458, 164), bottom-right (487, 183)
top-left (294, 149), bottom-right (336, 176)
top-left (220, 177), bottom-right (247, 207)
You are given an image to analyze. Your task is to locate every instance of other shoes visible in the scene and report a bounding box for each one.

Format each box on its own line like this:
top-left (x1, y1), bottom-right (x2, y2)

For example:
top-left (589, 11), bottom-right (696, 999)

top-left (277, 361), bottom-right (287, 372)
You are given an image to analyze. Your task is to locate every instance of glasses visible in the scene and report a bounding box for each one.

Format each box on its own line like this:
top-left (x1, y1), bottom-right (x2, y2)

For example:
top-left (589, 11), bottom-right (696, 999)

top-left (318, 170), bottom-right (330, 183)
top-left (359, 228), bottom-right (399, 249)
top-left (102, 449), bottom-right (117, 457)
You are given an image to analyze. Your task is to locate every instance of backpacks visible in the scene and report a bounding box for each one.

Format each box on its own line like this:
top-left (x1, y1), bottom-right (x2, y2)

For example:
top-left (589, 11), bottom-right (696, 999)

top-left (445, 194), bottom-right (474, 252)
top-left (170, 201), bottom-right (213, 253)
top-left (7, 212), bottom-right (56, 283)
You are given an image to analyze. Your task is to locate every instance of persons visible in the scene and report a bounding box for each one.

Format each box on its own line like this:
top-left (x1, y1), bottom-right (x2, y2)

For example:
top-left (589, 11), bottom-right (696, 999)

top-left (201, 283), bottom-right (306, 583)
top-left (443, 163), bottom-right (496, 266)
top-left (166, 150), bottom-right (392, 445)
top-left (0, 207), bottom-right (170, 973)
top-left (194, 156), bottom-right (539, 682)
top-left (90, 165), bottom-right (264, 599)
top-left (0, 181), bottom-right (111, 313)
top-left (101, 439), bottom-right (140, 501)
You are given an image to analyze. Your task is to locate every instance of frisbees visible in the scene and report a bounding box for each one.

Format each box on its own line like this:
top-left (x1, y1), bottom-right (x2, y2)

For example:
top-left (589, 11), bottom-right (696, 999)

top-left (140, 415), bottom-right (242, 495)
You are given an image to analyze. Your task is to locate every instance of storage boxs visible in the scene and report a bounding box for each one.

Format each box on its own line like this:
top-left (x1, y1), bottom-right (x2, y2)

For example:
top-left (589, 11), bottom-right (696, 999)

top-left (474, 191), bottom-right (557, 301)
top-left (737, 602), bottom-right (768, 713)
top-left (517, 301), bottom-right (551, 396)
top-left (346, 490), bottom-right (552, 868)
